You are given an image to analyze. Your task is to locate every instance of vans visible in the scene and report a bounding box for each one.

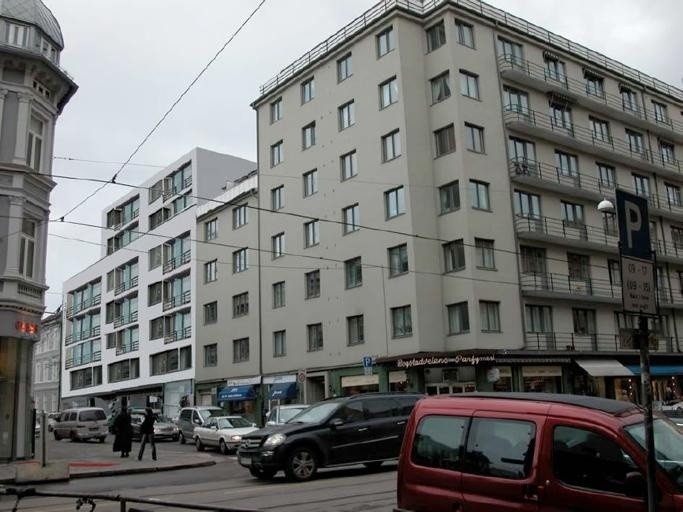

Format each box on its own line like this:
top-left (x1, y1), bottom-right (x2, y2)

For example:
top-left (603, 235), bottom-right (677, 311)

top-left (53, 408), bottom-right (110, 443)
top-left (397, 391), bottom-right (683, 512)
top-left (177, 405), bottom-right (228, 444)
top-left (237, 390), bottom-right (426, 481)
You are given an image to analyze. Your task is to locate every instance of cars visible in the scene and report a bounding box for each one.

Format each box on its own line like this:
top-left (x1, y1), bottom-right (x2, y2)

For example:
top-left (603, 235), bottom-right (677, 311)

top-left (129, 409), bottom-right (180, 441)
top-left (192, 416), bottom-right (260, 454)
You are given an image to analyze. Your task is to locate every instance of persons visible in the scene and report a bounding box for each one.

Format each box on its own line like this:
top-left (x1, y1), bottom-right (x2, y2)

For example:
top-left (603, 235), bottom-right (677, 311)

top-left (111, 406), bottom-right (133, 457)
top-left (618, 390), bottom-right (629, 402)
top-left (665, 387), bottom-right (673, 406)
top-left (138, 407), bottom-right (157, 461)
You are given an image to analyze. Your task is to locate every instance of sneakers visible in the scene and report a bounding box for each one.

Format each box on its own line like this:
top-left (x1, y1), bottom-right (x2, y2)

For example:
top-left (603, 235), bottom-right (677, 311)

top-left (121, 452), bottom-right (129, 457)
top-left (137, 456), bottom-right (157, 460)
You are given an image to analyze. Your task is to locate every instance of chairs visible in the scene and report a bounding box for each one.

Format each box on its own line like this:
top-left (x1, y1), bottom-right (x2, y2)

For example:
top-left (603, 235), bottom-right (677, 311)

top-left (552, 440), bottom-right (626, 492)
